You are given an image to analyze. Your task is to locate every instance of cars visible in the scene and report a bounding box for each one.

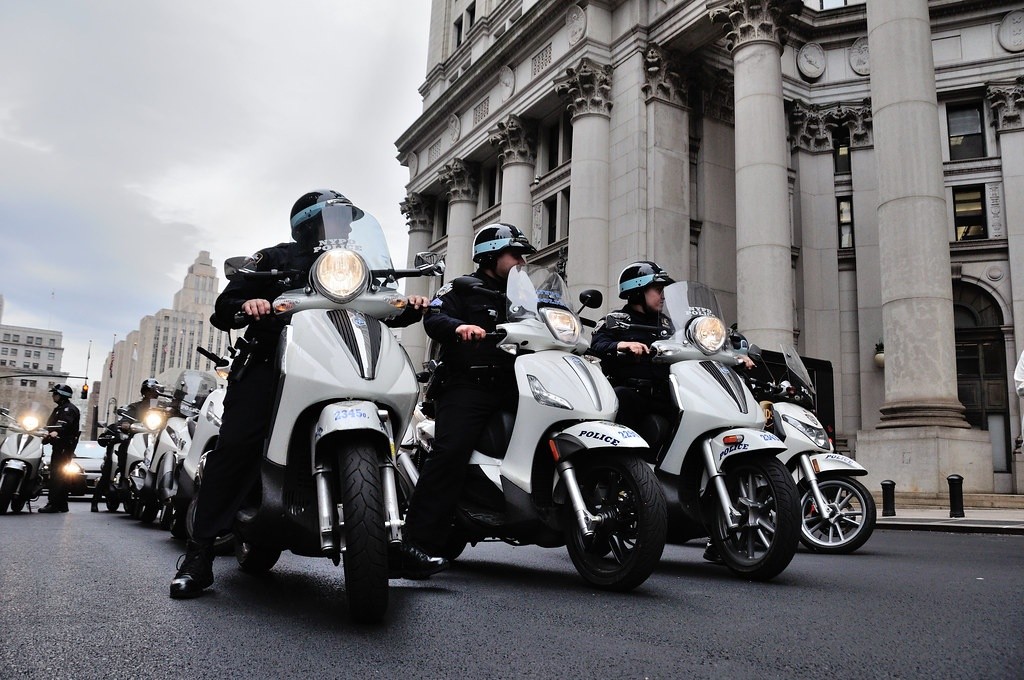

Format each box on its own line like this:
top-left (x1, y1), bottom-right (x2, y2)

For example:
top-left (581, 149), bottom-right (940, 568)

top-left (41, 440), bottom-right (118, 498)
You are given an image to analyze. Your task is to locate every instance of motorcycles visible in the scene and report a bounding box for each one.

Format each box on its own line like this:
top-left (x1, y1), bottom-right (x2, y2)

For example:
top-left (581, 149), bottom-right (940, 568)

top-left (0, 407), bottom-right (67, 515)
top-left (96, 205), bottom-right (876, 612)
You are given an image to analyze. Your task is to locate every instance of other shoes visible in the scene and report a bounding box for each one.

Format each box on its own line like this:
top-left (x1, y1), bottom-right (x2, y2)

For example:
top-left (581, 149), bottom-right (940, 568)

top-left (704, 541), bottom-right (725, 565)
top-left (91, 500), bottom-right (99, 512)
top-left (38, 498), bottom-right (69, 513)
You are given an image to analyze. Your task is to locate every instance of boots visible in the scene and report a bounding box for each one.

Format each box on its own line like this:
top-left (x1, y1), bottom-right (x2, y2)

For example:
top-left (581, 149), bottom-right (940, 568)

top-left (390, 544), bottom-right (452, 580)
top-left (169, 539), bottom-right (217, 598)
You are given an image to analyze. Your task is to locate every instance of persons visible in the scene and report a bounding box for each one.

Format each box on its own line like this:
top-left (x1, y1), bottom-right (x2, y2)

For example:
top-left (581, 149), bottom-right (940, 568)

top-left (170, 191), bottom-right (449, 597)
top-left (402, 223), bottom-right (537, 551)
top-left (38, 384), bottom-right (80, 513)
top-left (90, 421), bottom-right (119, 511)
top-left (590, 260), bottom-right (755, 563)
top-left (118, 378), bottom-right (160, 489)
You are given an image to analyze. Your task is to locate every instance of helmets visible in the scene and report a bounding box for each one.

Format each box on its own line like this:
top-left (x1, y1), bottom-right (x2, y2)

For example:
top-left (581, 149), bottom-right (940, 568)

top-left (140, 379), bottom-right (160, 394)
top-left (731, 331), bottom-right (749, 350)
top-left (48, 383), bottom-right (73, 398)
top-left (291, 189), bottom-right (364, 242)
top-left (618, 261), bottom-right (676, 300)
top-left (473, 223), bottom-right (538, 264)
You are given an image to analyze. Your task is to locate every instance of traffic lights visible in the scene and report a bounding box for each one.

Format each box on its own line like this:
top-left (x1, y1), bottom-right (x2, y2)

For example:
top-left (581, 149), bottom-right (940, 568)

top-left (81, 384), bottom-right (88, 399)
